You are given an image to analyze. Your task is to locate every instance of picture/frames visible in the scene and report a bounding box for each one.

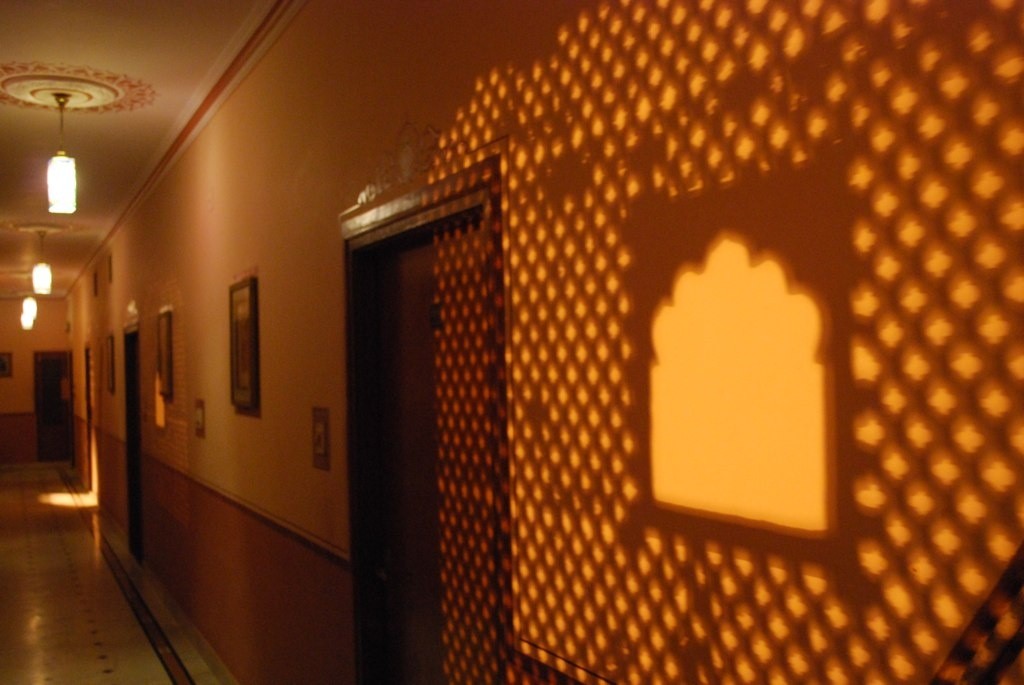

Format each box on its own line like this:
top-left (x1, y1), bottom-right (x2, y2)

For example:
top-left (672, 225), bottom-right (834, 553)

top-left (156, 310), bottom-right (173, 397)
top-left (0, 352), bottom-right (13, 376)
top-left (229, 277), bottom-right (259, 408)
top-left (105, 336), bottom-right (115, 394)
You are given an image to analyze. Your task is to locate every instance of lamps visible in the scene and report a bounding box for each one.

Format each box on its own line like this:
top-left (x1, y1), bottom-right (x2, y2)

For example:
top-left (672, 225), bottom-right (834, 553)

top-left (46, 93), bottom-right (76, 213)
top-left (32, 230), bottom-right (52, 295)
